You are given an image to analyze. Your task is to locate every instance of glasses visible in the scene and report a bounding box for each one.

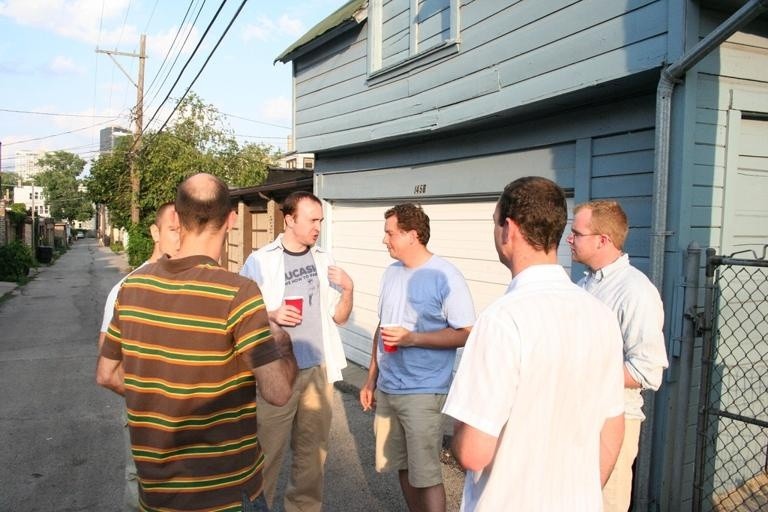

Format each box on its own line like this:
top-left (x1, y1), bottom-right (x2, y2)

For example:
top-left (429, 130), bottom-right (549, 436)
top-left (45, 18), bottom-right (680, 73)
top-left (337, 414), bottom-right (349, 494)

top-left (572, 232), bottom-right (601, 238)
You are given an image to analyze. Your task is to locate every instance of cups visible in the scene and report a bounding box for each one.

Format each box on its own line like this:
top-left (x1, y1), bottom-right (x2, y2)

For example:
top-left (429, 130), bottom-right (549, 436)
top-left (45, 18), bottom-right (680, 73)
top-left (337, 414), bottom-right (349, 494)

top-left (381, 324), bottom-right (400, 352)
top-left (285, 296), bottom-right (303, 322)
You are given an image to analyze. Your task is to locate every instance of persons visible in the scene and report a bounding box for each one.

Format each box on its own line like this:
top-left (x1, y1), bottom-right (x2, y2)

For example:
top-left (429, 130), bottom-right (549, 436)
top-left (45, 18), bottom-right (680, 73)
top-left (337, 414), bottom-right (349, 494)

top-left (237, 191), bottom-right (354, 512)
top-left (566, 201), bottom-right (669, 512)
top-left (98, 202), bottom-right (182, 511)
top-left (360, 203), bottom-right (476, 511)
top-left (441, 176), bottom-right (625, 512)
top-left (95, 172), bottom-right (298, 512)
